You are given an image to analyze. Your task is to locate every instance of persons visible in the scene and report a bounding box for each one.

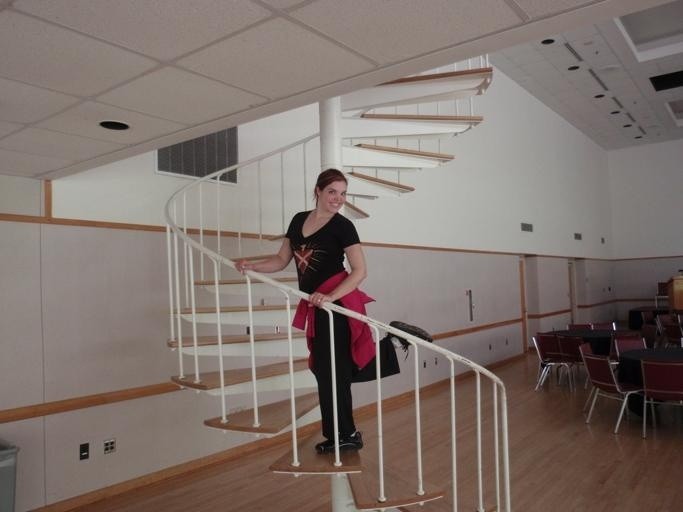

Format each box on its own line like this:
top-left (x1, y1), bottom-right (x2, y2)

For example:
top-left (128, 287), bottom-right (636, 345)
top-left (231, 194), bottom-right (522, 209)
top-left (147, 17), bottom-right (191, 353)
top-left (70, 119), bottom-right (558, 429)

top-left (233, 168), bottom-right (432, 454)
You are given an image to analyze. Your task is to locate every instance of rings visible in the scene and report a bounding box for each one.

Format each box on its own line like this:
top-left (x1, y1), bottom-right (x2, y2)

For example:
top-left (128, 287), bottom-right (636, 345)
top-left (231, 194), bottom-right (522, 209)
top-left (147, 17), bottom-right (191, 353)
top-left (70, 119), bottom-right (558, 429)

top-left (316, 298), bottom-right (320, 302)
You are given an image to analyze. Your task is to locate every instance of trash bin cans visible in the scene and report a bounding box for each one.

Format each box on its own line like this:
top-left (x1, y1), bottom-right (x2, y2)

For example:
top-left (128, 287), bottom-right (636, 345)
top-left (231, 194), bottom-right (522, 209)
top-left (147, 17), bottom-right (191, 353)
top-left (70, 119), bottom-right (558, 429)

top-left (0, 439), bottom-right (20, 512)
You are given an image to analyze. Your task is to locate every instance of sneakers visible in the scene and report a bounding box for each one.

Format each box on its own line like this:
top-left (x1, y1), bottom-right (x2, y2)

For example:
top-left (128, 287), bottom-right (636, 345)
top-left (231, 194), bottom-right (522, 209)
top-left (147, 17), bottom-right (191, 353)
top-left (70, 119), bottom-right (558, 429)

top-left (386, 320), bottom-right (433, 361)
top-left (315, 431), bottom-right (364, 454)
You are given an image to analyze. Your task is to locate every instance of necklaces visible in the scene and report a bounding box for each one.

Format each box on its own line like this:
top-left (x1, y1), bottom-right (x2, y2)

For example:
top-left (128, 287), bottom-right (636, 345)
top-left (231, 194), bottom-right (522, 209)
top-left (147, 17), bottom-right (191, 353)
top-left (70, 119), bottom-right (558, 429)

top-left (308, 211), bottom-right (330, 233)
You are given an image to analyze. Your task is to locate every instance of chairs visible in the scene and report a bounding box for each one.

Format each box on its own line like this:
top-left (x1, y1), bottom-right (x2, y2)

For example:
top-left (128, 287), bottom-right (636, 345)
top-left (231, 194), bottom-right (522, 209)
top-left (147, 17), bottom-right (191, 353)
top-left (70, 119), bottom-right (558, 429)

top-left (531, 282), bottom-right (683, 439)
